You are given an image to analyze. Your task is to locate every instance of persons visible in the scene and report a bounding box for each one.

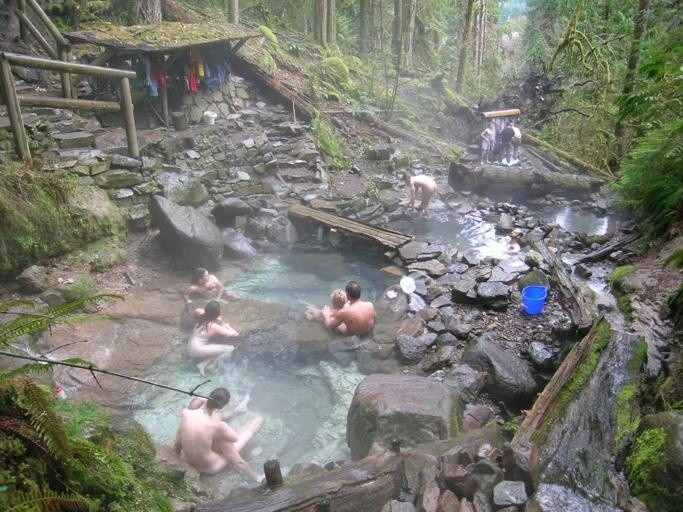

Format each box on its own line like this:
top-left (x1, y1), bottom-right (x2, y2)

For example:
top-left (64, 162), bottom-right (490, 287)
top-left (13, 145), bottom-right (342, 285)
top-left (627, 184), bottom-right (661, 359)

top-left (401, 172), bottom-right (437, 215)
top-left (180, 386), bottom-right (265, 483)
top-left (186, 300), bottom-right (239, 377)
top-left (321, 280), bottom-right (376, 336)
top-left (173, 397), bottom-right (260, 484)
top-left (303, 287), bottom-right (346, 330)
top-left (479, 121), bottom-right (522, 165)
top-left (182, 266), bottom-right (240, 303)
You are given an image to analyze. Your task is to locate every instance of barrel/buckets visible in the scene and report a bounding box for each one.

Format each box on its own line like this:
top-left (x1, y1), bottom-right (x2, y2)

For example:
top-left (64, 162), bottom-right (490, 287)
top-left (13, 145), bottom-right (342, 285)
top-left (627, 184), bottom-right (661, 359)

top-left (203, 111), bottom-right (217, 125)
top-left (522, 285), bottom-right (548, 315)
